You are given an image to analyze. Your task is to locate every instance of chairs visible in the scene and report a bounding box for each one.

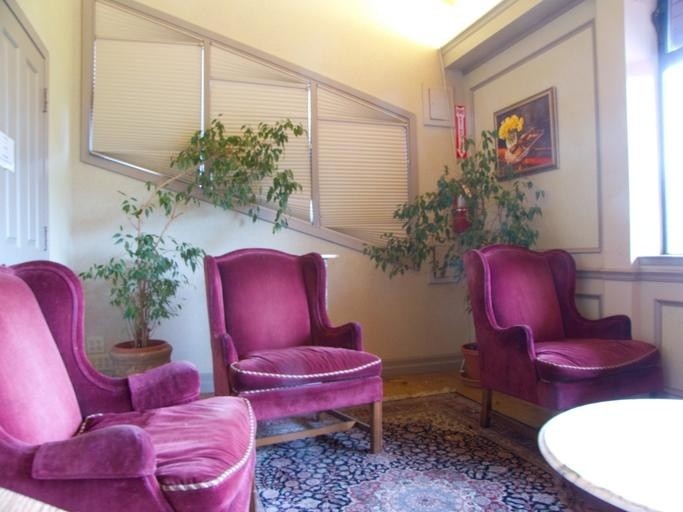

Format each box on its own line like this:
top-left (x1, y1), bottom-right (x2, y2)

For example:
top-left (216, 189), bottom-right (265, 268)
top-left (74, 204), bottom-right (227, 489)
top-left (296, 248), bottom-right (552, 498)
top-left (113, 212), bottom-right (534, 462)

top-left (460, 240), bottom-right (667, 431)
top-left (0, 258), bottom-right (259, 511)
top-left (200, 245), bottom-right (386, 455)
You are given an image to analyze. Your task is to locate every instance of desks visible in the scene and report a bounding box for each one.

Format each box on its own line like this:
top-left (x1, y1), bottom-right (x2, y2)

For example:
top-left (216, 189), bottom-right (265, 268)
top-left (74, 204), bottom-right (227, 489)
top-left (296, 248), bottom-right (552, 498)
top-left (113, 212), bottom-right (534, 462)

top-left (535, 395), bottom-right (682, 511)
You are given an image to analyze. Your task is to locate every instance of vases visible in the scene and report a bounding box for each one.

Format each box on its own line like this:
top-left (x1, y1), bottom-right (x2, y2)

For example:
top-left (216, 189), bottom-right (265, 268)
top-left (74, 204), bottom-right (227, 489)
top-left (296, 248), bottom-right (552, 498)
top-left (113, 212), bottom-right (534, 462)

top-left (505, 130), bottom-right (519, 153)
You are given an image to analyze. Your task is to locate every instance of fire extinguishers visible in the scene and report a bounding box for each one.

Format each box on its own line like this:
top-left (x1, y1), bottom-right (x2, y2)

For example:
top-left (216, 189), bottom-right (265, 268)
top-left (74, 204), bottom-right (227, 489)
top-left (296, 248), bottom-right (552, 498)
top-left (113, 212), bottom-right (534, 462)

top-left (451, 179), bottom-right (472, 234)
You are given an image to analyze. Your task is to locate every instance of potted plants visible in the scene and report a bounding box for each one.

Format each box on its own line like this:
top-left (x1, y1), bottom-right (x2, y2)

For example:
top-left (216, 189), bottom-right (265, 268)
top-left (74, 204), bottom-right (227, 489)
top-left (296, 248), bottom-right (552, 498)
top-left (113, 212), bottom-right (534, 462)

top-left (359, 127), bottom-right (547, 389)
top-left (74, 108), bottom-right (310, 379)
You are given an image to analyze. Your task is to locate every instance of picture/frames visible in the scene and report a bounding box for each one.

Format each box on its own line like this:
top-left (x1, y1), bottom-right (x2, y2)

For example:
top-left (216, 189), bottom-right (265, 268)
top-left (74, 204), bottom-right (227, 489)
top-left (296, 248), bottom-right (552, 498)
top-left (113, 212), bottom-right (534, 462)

top-left (491, 85), bottom-right (559, 182)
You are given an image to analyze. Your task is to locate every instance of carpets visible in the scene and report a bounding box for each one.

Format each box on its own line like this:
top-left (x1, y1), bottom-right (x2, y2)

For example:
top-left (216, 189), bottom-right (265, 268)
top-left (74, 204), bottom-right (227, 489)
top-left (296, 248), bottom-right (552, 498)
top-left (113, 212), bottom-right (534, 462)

top-left (253, 390), bottom-right (607, 511)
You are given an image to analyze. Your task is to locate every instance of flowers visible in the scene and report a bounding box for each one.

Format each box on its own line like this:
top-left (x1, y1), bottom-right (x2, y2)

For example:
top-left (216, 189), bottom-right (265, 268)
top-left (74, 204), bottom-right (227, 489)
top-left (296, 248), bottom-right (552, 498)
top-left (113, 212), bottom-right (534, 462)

top-left (497, 113), bottom-right (525, 142)
